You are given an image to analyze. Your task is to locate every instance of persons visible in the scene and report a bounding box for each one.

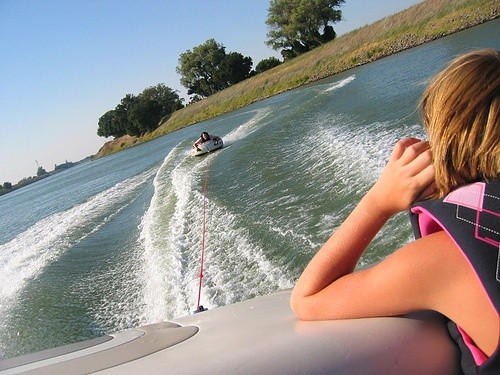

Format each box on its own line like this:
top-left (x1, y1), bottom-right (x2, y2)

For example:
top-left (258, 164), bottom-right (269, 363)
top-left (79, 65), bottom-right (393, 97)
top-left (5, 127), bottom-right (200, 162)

top-left (290, 49), bottom-right (500, 375)
top-left (192, 131), bottom-right (219, 148)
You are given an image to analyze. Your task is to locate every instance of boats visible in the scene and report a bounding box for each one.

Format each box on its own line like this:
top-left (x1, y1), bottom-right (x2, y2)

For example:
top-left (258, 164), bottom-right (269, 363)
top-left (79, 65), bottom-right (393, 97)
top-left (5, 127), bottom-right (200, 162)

top-left (191, 138), bottom-right (223, 156)
